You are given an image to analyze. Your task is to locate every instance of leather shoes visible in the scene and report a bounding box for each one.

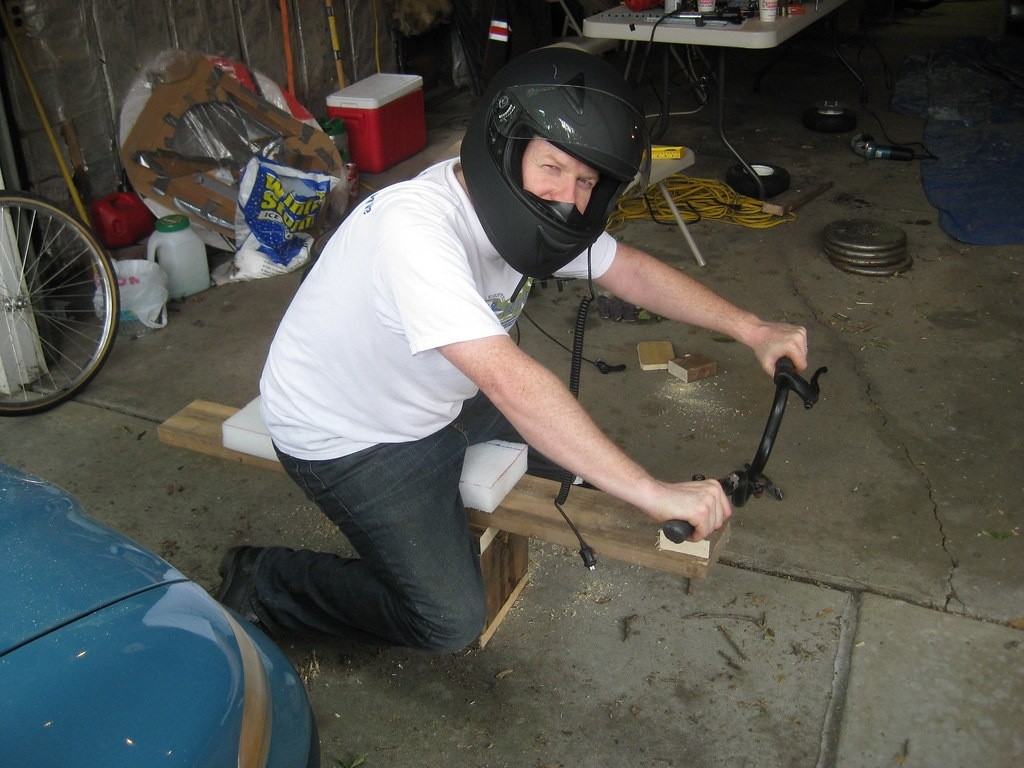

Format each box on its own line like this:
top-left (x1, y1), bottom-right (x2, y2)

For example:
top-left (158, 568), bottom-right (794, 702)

top-left (214, 545), bottom-right (279, 642)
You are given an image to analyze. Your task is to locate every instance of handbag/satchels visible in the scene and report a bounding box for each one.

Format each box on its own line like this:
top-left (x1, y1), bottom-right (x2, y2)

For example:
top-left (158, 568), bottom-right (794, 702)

top-left (451, 29), bottom-right (472, 89)
top-left (93, 258), bottom-right (168, 329)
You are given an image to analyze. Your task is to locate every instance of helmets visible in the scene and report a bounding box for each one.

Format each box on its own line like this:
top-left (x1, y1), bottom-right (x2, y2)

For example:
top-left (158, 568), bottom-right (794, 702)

top-left (459, 48), bottom-right (653, 278)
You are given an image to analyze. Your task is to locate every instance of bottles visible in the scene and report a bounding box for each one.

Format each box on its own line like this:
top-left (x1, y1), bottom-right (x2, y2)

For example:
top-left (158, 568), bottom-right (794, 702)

top-left (147, 213), bottom-right (210, 297)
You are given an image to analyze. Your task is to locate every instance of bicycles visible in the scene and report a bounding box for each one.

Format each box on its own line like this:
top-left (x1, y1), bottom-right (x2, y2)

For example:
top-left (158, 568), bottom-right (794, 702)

top-left (1, 189), bottom-right (122, 418)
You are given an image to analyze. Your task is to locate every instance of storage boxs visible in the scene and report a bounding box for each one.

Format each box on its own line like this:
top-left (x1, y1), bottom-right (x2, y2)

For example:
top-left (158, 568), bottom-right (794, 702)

top-left (325, 73), bottom-right (427, 174)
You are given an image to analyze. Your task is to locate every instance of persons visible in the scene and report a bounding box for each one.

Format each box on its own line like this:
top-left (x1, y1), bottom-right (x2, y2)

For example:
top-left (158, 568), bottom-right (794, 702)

top-left (210, 49), bottom-right (812, 651)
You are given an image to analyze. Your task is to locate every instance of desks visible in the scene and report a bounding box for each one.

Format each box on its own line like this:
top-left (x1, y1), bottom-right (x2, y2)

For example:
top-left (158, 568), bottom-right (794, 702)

top-left (584, 0), bottom-right (845, 200)
